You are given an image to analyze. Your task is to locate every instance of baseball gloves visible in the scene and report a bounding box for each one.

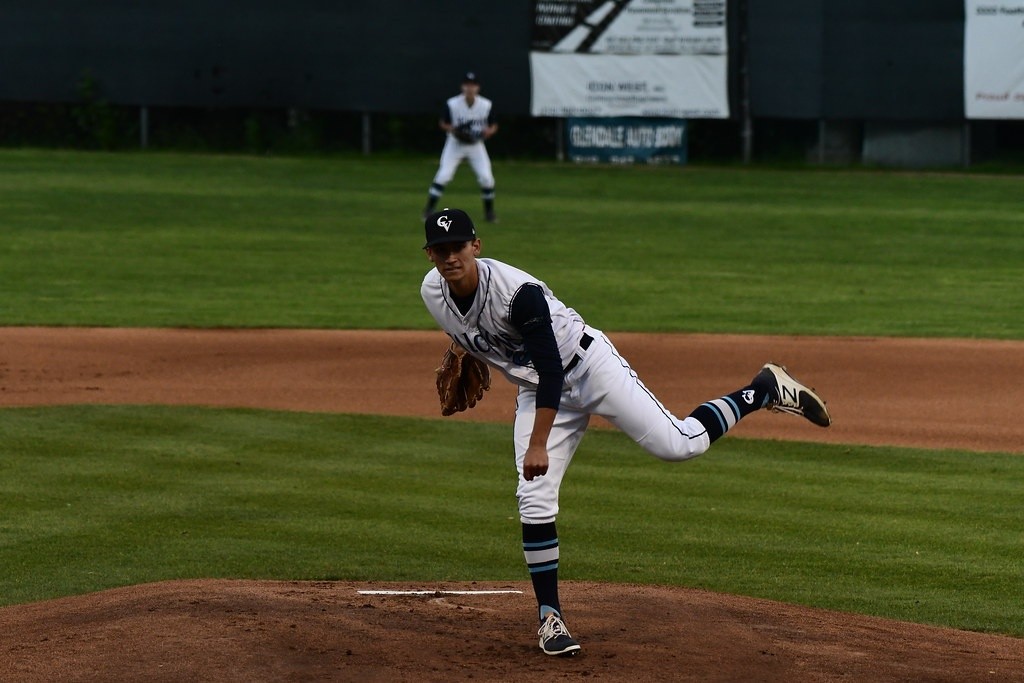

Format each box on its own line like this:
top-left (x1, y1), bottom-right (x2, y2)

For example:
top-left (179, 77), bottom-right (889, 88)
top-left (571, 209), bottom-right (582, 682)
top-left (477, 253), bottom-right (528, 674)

top-left (436, 340), bottom-right (492, 417)
top-left (453, 122), bottom-right (476, 143)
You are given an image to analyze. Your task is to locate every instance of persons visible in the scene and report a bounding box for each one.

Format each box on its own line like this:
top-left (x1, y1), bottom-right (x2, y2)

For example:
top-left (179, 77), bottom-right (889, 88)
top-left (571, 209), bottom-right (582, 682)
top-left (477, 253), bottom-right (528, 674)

top-left (420, 209), bottom-right (831, 654)
top-left (424, 72), bottom-right (498, 221)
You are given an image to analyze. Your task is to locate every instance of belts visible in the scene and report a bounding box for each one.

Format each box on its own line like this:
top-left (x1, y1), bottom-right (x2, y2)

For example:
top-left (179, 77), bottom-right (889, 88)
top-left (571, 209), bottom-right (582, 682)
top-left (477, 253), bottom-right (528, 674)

top-left (562, 332), bottom-right (594, 377)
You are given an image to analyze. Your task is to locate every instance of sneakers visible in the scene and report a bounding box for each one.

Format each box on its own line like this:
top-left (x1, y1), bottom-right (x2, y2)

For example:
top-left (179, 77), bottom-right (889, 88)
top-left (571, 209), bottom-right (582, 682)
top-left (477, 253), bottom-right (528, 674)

top-left (538, 615), bottom-right (581, 655)
top-left (751, 362), bottom-right (833, 427)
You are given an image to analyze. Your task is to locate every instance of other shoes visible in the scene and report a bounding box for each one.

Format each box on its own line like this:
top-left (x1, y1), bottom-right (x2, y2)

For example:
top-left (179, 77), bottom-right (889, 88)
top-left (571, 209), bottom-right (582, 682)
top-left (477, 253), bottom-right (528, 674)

top-left (488, 214), bottom-right (499, 223)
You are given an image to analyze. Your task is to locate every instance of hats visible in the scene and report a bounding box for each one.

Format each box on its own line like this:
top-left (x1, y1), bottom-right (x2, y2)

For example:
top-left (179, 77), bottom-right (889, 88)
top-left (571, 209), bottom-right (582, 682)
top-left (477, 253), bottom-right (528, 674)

top-left (423, 207), bottom-right (476, 251)
top-left (462, 71), bottom-right (480, 86)
